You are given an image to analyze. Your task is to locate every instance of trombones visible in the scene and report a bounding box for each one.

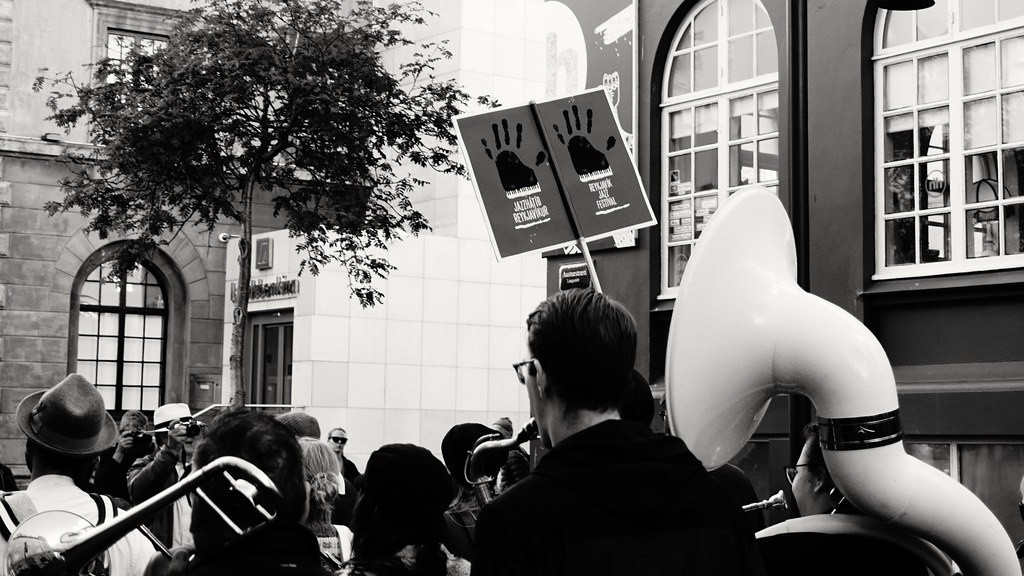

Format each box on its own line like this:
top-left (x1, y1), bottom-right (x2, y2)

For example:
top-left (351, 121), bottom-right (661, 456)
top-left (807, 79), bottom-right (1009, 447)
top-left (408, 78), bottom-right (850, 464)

top-left (3, 455), bottom-right (287, 576)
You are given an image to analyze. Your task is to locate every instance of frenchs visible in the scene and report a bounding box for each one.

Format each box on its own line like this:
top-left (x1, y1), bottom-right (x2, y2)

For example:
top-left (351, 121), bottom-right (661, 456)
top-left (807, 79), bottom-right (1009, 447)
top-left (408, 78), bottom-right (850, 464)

top-left (663, 185), bottom-right (1023, 576)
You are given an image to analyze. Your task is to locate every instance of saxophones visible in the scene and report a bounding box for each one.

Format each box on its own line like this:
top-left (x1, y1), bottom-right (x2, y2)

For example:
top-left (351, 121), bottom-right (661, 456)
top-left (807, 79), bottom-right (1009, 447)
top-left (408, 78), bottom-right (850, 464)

top-left (464, 417), bottom-right (539, 507)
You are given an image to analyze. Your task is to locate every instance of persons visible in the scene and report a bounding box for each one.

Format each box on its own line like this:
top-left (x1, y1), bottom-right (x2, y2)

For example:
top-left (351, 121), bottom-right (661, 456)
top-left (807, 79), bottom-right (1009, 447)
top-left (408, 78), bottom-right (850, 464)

top-left (0, 374), bottom-right (157, 576)
top-left (470, 289), bottom-right (757, 576)
top-left (756, 421), bottom-right (1024, 576)
top-left (96, 403), bottom-right (532, 576)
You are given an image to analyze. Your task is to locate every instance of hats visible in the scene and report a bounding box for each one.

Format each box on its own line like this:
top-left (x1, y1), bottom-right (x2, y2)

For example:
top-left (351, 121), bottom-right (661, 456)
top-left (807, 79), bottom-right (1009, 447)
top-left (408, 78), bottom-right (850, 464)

top-left (15, 373), bottom-right (119, 454)
top-left (141, 403), bottom-right (207, 435)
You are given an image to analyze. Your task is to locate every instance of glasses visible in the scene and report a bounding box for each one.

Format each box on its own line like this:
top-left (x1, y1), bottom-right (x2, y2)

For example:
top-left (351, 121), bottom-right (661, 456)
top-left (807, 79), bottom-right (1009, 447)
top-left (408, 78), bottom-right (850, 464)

top-left (328, 436), bottom-right (348, 444)
top-left (784, 464), bottom-right (809, 485)
top-left (513, 360), bottom-right (534, 385)
